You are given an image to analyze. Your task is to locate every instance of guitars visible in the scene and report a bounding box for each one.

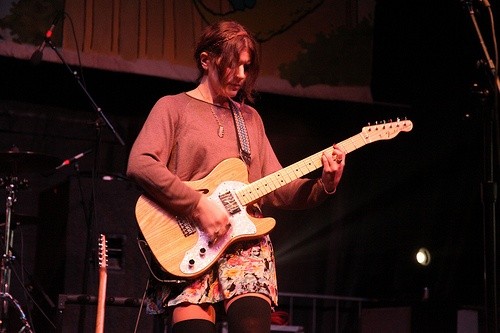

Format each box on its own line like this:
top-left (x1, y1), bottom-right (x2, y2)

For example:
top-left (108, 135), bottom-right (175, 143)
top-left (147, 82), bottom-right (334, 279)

top-left (133, 117), bottom-right (415, 283)
top-left (95, 233), bottom-right (109, 333)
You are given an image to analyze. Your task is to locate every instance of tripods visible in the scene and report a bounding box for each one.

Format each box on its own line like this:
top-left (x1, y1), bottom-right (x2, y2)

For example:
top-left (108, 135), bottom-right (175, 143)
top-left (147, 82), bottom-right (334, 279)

top-left (0, 185), bottom-right (33, 333)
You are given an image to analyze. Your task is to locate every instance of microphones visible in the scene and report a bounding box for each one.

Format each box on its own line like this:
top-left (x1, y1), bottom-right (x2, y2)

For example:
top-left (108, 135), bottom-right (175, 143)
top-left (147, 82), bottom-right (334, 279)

top-left (55, 150), bottom-right (92, 170)
top-left (30, 13), bottom-right (63, 64)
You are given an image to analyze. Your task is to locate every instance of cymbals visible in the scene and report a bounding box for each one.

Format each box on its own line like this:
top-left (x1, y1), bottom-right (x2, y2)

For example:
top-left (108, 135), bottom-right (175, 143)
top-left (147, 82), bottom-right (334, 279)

top-left (0, 148), bottom-right (70, 177)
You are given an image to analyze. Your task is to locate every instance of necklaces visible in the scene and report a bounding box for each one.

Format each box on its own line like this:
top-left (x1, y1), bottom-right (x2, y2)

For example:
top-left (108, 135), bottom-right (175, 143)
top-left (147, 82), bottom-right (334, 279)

top-left (197, 84), bottom-right (230, 139)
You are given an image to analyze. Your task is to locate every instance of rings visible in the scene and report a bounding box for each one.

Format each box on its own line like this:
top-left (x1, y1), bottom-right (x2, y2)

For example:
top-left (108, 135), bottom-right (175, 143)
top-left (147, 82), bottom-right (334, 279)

top-left (214, 228), bottom-right (221, 234)
top-left (333, 158), bottom-right (342, 165)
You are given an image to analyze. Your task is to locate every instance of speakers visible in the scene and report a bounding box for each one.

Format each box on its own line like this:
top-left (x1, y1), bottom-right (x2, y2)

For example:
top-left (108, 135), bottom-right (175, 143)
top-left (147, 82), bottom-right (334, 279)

top-left (56, 176), bottom-right (163, 333)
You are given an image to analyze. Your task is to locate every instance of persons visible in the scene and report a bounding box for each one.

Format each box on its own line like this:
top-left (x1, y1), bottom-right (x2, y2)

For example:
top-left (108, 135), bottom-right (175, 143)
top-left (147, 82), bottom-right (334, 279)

top-left (127, 19), bottom-right (345, 333)
